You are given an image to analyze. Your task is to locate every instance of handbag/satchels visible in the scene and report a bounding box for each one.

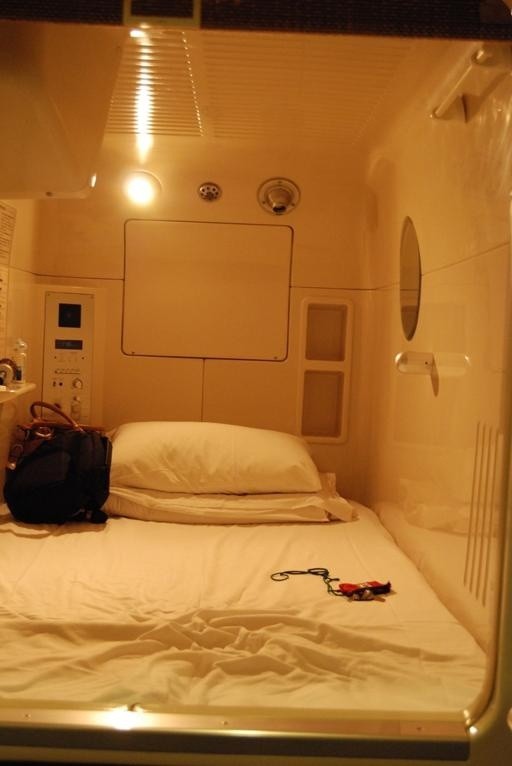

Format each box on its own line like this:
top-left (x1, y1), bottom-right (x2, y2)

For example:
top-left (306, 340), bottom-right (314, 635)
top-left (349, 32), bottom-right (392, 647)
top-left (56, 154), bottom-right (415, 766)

top-left (4, 402), bottom-right (112, 523)
top-left (339, 581), bottom-right (390, 600)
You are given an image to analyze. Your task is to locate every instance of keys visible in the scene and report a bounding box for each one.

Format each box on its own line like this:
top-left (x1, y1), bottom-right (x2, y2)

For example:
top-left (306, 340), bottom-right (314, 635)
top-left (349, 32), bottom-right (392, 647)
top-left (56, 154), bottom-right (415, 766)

top-left (360, 589), bottom-right (386, 602)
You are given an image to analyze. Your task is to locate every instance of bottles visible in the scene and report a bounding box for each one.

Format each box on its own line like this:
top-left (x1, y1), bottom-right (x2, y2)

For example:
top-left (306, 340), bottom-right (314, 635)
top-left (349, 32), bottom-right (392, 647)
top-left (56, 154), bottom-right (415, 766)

top-left (11, 336), bottom-right (28, 389)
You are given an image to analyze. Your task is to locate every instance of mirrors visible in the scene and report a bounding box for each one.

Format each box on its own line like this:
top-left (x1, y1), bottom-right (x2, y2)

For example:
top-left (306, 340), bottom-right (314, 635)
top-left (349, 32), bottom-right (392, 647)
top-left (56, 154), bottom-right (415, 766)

top-left (397, 216), bottom-right (422, 341)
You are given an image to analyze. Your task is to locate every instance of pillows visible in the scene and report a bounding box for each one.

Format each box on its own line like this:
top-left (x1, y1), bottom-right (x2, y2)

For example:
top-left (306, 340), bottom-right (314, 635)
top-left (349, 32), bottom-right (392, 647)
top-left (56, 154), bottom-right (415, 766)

top-left (108, 420), bottom-right (323, 494)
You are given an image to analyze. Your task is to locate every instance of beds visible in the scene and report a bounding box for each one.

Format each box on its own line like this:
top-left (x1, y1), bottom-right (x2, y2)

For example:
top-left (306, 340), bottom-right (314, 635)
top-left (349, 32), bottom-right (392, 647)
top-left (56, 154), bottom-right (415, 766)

top-left (1, 496), bottom-right (489, 713)
